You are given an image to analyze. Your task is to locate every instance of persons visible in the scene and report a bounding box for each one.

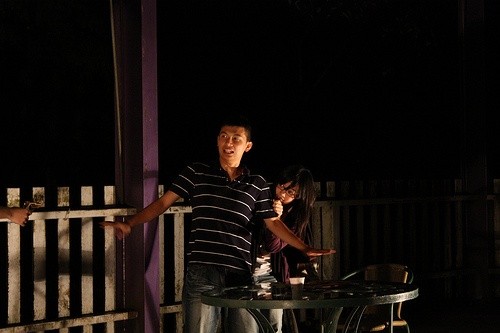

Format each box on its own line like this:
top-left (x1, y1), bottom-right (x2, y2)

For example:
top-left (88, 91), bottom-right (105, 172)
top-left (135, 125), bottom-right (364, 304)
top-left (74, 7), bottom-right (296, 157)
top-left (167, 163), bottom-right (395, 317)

top-left (250, 167), bottom-right (317, 333)
top-left (100, 119), bottom-right (336, 333)
top-left (0, 206), bottom-right (31, 227)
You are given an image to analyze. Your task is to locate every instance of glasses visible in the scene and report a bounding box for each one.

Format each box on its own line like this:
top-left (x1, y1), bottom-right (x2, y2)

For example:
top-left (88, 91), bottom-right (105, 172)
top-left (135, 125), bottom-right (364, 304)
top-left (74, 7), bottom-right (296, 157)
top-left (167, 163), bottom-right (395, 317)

top-left (277, 184), bottom-right (298, 198)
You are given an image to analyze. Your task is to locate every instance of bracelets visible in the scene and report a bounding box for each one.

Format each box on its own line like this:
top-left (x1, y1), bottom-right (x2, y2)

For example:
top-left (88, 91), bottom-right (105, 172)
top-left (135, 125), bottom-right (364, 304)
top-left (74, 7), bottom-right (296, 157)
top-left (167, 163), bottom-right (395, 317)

top-left (123, 220), bottom-right (132, 227)
top-left (302, 247), bottom-right (314, 254)
top-left (8, 208), bottom-right (13, 219)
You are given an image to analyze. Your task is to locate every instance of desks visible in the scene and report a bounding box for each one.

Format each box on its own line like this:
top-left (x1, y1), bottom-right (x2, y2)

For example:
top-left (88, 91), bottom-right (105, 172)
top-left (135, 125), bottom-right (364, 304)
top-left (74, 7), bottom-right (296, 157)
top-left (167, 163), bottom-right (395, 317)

top-left (201, 278), bottom-right (419, 333)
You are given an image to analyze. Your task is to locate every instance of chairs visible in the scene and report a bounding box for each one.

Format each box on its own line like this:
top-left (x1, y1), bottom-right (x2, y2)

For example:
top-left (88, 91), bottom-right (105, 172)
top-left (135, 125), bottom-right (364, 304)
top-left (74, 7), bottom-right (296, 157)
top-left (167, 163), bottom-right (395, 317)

top-left (321, 264), bottom-right (415, 333)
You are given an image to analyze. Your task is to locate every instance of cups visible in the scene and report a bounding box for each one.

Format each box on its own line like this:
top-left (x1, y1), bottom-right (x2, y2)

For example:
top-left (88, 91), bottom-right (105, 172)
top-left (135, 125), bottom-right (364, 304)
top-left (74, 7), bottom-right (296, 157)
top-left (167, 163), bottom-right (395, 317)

top-left (289, 276), bottom-right (305, 296)
